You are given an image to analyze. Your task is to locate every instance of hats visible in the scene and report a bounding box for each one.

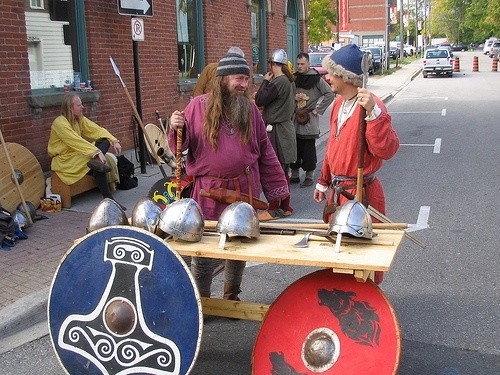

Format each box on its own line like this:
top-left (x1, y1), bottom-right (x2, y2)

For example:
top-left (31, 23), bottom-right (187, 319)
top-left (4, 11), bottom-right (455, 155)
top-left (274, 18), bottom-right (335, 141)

top-left (215, 46), bottom-right (250, 76)
top-left (321, 44), bottom-right (368, 87)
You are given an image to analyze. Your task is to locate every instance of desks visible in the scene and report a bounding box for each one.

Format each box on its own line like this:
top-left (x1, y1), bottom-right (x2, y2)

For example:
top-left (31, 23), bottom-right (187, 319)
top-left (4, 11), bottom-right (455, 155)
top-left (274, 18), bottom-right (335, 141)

top-left (74, 214), bottom-right (404, 323)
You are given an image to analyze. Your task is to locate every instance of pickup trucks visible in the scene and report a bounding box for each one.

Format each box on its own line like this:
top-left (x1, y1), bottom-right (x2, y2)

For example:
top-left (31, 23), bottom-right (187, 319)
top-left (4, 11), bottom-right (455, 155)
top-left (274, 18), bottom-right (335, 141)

top-left (422, 48), bottom-right (453, 79)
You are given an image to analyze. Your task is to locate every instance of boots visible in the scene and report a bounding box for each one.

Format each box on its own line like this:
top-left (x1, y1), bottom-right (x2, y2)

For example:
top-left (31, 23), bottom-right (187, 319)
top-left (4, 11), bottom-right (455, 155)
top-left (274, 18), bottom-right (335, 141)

top-left (223, 260), bottom-right (246, 321)
top-left (290, 170), bottom-right (300, 183)
top-left (299, 170), bottom-right (314, 188)
top-left (191, 262), bottom-right (214, 320)
top-left (87, 142), bottom-right (112, 172)
top-left (95, 174), bottom-right (126, 210)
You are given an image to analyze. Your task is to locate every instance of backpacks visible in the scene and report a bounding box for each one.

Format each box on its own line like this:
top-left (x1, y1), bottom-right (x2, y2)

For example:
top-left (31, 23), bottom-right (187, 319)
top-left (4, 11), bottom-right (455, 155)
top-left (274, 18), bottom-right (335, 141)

top-left (116, 155), bottom-right (138, 190)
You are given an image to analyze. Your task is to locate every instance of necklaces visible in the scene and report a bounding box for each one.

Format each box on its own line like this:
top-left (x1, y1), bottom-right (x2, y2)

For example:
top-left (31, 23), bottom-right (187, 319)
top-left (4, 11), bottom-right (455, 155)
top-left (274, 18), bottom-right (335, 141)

top-left (349, 92), bottom-right (358, 100)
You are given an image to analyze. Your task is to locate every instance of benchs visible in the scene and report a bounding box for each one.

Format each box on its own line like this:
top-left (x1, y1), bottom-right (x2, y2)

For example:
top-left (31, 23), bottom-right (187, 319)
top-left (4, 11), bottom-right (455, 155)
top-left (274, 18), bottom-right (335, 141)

top-left (49, 169), bottom-right (102, 209)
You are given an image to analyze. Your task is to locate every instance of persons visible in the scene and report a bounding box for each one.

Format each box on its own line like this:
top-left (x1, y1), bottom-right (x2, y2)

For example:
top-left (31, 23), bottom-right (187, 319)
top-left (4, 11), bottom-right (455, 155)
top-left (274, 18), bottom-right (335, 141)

top-left (47, 94), bottom-right (127, 211)
top-left (168, 49), bottom-right (336, 321)
top-left (313, 44), bottom-right (399, 290)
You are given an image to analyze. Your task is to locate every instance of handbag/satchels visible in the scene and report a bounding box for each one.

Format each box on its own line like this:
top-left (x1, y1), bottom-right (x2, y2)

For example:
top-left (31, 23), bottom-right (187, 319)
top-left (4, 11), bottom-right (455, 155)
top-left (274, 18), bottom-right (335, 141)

top-left (296, 110), bottom-right (310, 126)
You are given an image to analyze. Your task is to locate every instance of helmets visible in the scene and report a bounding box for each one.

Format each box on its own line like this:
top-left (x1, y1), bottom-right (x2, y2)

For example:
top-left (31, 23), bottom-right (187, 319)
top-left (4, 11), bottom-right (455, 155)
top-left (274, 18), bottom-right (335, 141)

top-left (266, 49), bottom-right (288, 65)
top-left (216, 201), bottom-right (260, 250)
top-left (11, 201), bottom-right (36, 228)
top-left (159, 198), bottom-right (205, 242)
top-left (327, 200), bottom-right (372, 252)
top-left (86, 198), bottom-right (130, 234)
top-left (132, 196), bottom-right (164, 238)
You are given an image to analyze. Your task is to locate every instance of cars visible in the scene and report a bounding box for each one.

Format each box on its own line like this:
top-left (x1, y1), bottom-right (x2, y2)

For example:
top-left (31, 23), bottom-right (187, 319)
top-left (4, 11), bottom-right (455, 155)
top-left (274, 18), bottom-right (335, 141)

top-left (435, 38), bottom-right (500, 58)
top-left (307, 51), bottom-right (335, 84)
top-left (361, 43), bottom-right (421, 75)
